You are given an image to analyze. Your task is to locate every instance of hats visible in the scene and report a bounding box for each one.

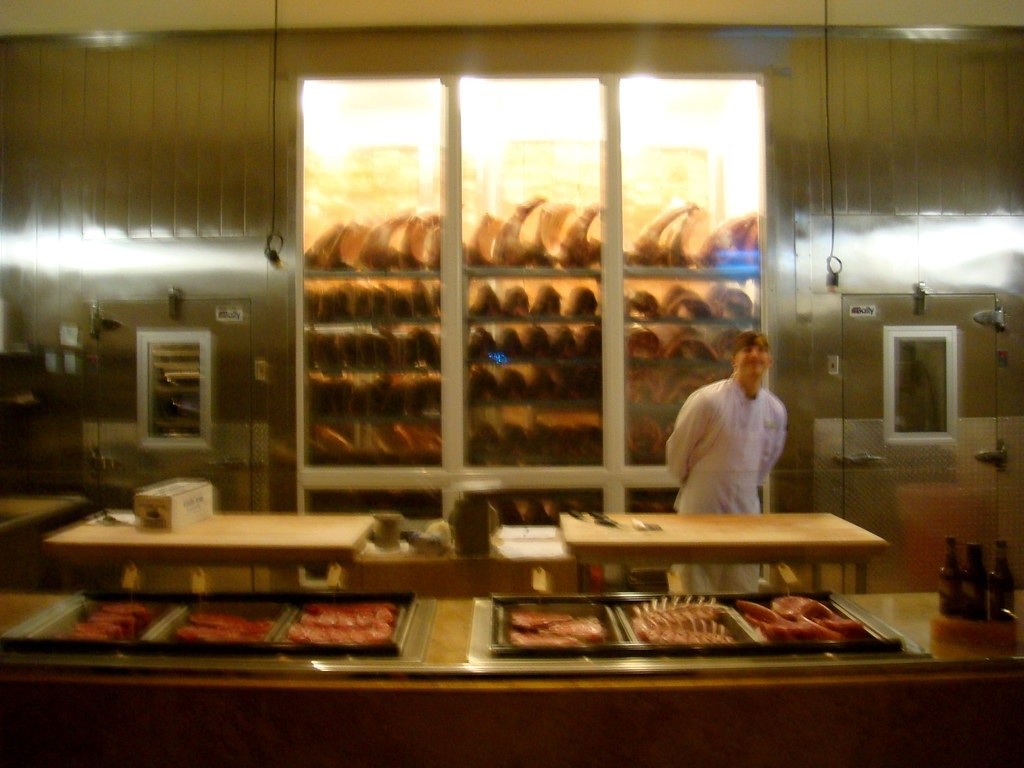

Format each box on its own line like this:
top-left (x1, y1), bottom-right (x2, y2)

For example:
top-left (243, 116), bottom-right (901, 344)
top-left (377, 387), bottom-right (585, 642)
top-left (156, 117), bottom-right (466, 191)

top-left (733, 331), bottom-right (768, 355)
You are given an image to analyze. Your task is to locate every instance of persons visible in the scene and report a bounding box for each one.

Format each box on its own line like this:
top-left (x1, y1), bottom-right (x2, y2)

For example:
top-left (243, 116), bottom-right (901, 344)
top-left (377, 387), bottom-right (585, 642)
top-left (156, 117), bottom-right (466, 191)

top-left (667, 332), bottom-right (787, 595)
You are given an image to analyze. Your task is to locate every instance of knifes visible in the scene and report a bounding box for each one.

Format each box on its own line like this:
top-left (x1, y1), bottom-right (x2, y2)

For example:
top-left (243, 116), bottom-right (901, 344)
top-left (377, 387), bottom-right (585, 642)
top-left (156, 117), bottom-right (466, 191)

top-left (589, 511), bottom-right (617, 528)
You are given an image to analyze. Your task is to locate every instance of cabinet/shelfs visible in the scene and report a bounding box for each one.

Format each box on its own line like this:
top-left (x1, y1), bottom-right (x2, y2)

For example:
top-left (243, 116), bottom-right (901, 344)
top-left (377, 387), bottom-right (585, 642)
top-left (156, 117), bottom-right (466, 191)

top-left (294, 71), bottom-right (772, 527)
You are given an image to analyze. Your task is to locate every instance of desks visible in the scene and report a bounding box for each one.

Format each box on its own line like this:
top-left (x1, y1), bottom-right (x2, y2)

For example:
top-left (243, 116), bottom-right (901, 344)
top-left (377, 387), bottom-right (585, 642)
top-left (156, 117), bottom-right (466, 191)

top-left (42, 506), bottom-right (378, 596)
top-left (0, 589), bottom-right (1024, 768)
top-left (558, 510), bottom-right (890, 598)
top-left (337, 524), bottom-right (579, 598)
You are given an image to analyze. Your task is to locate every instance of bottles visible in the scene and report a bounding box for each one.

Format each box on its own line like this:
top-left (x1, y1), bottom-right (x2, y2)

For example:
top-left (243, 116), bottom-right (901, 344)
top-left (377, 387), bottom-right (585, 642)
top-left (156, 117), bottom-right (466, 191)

top-left (939, 537), bottom-right (960, 615)
top-left (962, 544), bottom-right (987, 620)
top-left (989, 540), bottom-right (1014, 621)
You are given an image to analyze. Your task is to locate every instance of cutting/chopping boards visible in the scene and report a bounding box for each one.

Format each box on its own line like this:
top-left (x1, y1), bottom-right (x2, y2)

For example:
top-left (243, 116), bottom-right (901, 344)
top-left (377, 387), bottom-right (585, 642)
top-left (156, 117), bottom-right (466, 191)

top-left (558, 511), bottom-right (884, 545)
